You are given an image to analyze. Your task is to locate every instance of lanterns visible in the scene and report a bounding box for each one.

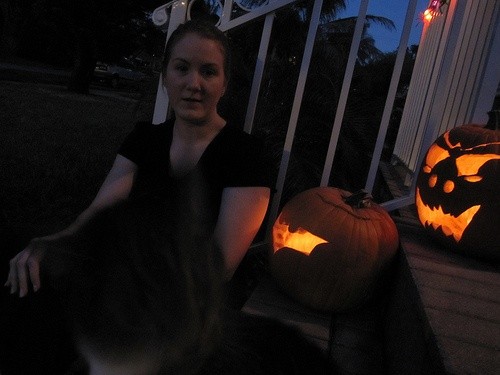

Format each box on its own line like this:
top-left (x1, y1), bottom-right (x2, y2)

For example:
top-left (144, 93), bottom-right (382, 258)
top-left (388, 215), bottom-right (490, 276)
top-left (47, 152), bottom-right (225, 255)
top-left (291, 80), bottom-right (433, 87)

top-left (269, 187), bottom-right (401, 319)
top-left (413, 123), bottom-right (500, 263)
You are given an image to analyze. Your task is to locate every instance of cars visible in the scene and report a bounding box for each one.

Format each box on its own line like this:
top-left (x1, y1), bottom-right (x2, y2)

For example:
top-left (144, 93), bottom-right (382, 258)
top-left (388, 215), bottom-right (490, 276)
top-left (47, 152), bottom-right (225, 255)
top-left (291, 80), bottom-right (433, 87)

top-left (93, 60), bottom-right (120, 85)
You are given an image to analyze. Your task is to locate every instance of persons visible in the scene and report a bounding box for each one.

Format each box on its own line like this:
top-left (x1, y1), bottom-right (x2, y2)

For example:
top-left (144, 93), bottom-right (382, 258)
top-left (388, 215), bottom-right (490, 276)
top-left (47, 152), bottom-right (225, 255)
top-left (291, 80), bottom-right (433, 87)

top-left (0, 20), bottom-right (274, 375)
top-left (53, 206), bottom-right (224, 374)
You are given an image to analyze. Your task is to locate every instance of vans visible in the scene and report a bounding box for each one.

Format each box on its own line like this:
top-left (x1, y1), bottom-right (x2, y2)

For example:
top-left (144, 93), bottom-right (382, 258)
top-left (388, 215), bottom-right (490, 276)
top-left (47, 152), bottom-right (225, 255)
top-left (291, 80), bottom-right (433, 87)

top-left (107, 56), bottom-right (161, 93)
